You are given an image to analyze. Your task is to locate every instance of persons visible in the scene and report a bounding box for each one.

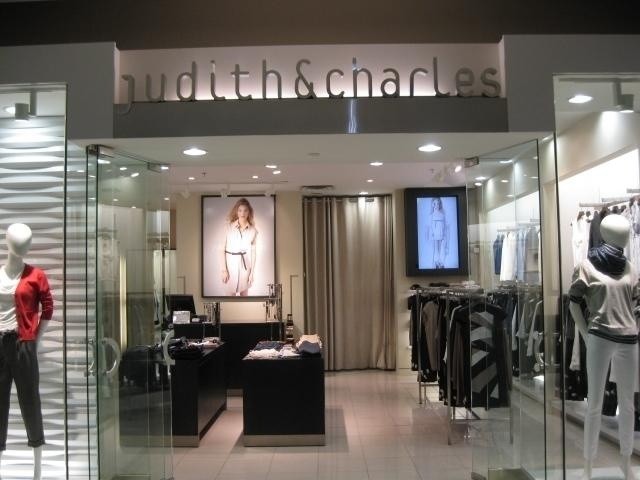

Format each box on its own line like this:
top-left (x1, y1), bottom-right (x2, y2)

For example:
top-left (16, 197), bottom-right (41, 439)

top-left (1, 221), bottom-right (55, 480)
top-left (221, 197), bottom-right (258, 296)
top-left (569, 211), bottom-right (640, 480)
top-left (426, 197), bottom-right (449, 268)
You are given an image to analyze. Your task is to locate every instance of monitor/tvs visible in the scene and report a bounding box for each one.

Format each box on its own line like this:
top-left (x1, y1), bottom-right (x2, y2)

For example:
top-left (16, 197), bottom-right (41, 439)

top-left (165, 294), bottom-right (196, 315)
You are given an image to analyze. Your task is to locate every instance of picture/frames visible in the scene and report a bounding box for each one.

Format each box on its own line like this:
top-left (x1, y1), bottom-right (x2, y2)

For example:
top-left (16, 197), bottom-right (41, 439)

top-left (404, 187), bottom-right (470, 277)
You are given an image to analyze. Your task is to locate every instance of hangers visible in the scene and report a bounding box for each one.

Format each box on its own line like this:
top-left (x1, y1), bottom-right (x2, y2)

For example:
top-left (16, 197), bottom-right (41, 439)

top-left (420, 286), bottom-right (466, 297)
